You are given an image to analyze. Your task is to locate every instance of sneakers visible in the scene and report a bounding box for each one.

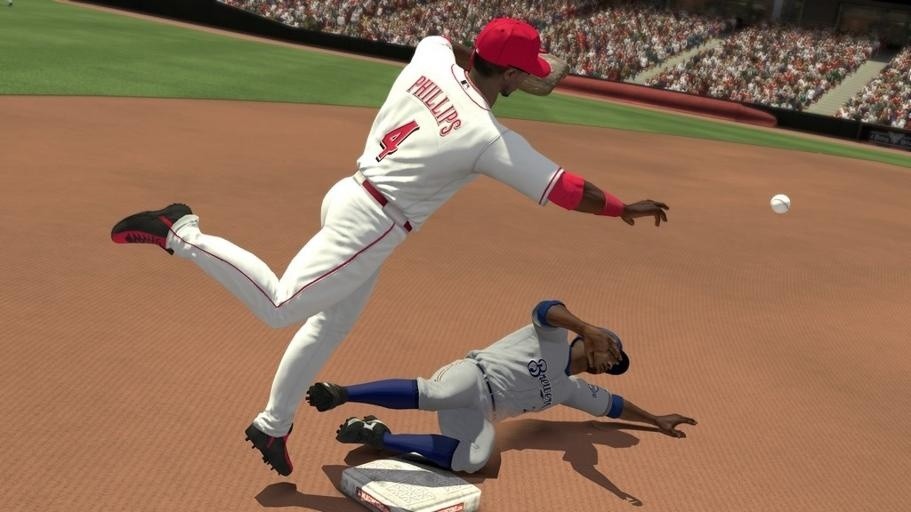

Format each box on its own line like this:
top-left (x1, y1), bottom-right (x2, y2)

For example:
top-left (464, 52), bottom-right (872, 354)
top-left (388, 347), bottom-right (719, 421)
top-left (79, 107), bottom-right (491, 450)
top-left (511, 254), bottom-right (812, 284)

top-left (245, 423), bottom-right (293, 476)
top-left (305, 382), bottom-right (345, 412)
top-left (336, 415), bottom-right (391, 443)
top-left (110, 203), bottom-right (192, 255)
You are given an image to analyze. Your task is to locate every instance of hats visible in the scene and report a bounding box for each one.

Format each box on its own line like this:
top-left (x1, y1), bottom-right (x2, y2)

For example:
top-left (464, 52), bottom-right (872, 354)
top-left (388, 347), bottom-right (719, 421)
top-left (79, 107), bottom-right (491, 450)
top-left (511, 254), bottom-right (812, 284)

top-left (570, 328), bottom-right (629, 376)
top-left (475, 18), bottom-right (550, 78)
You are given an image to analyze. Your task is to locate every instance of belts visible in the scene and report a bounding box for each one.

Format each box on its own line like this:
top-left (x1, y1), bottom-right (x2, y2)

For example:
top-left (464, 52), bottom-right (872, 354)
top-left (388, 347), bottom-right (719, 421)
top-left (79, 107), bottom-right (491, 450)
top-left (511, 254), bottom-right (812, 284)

top-left (362, 180), bottom-right (413, 232)
top-left (477, 363), bottom-right (496, 413)
